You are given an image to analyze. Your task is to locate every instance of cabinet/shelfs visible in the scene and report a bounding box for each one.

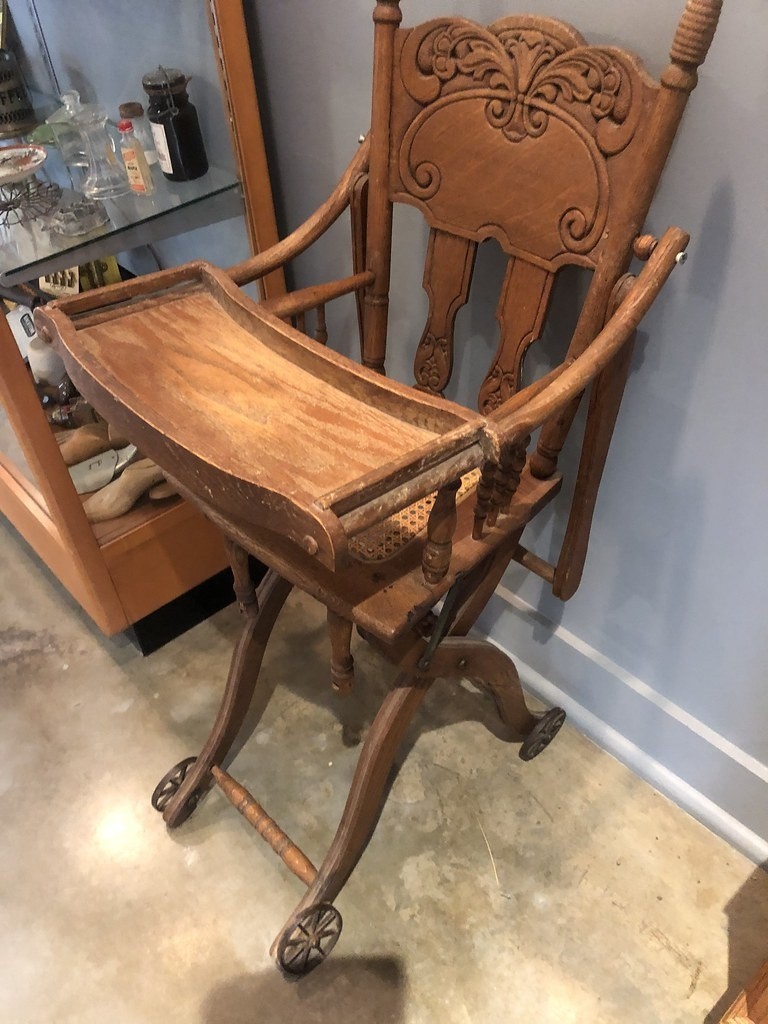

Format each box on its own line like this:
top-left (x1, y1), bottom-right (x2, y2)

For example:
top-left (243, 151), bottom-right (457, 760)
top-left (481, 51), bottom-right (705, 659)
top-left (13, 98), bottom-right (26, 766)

top-left (0, 0), bottom-right (294, 653)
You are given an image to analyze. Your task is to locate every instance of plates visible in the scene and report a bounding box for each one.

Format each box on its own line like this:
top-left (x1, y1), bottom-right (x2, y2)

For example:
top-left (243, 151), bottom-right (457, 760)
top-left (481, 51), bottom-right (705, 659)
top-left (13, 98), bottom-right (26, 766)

top-left (0, 144), bottom-right (48, 186)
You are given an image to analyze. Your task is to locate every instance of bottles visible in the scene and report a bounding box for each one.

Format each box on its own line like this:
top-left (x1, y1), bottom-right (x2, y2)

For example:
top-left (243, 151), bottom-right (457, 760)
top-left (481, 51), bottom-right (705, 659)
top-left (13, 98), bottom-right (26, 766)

top-left (142, 64), bottom-right (210, 182)
top-left (119, 102), bottom-right (159, 166)
top-left (117, 119), bottom-right (157, 197)
